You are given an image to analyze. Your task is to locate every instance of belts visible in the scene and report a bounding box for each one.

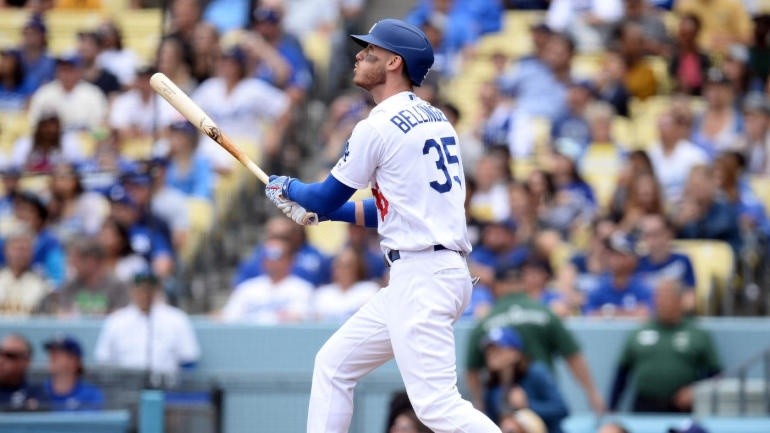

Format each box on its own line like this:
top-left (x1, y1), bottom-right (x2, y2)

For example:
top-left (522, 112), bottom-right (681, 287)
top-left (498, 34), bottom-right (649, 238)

top-left (384, 244), bottom-right (446, 267)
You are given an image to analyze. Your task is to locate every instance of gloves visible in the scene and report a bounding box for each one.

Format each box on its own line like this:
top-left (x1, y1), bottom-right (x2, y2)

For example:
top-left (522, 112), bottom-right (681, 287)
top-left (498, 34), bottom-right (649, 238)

top-left (264, 175), bottom-right (298, 208)
top-left (281, 203), bottom-right (319, 228)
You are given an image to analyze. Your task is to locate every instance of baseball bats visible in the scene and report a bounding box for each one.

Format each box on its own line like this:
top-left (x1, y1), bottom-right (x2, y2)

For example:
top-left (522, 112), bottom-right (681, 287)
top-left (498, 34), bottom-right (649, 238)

top-left (148, 71), bottom-right (315, 226)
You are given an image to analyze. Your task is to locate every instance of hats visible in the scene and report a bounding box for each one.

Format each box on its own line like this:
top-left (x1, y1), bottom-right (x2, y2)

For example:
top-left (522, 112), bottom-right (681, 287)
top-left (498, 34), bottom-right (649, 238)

top-left (44, 335), bottom-right (84, 359)
top-left (5, 0), bottom-right (283, 260)
top-left (476, 327), bottom-right (519, 355)
top-left (131, 269), bottom-right (156, 284)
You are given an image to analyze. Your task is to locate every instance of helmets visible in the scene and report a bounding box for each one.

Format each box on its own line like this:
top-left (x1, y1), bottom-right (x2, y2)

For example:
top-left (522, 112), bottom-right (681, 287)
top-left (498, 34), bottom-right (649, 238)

top-left (350, 19), bottom-right (435, 87)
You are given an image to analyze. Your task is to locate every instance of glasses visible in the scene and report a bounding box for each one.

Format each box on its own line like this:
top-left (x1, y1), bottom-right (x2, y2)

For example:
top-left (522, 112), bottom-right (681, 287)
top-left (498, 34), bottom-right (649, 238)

top-left (0, 350), bottom-right (21, 361)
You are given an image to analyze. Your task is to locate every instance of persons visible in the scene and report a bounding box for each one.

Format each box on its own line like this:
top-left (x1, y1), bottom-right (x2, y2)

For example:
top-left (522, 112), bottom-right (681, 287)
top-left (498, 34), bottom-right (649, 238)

top-left (0, 0), bottom-right (770, 433)
top-left (266, 17), bottom-right (503, 433)
